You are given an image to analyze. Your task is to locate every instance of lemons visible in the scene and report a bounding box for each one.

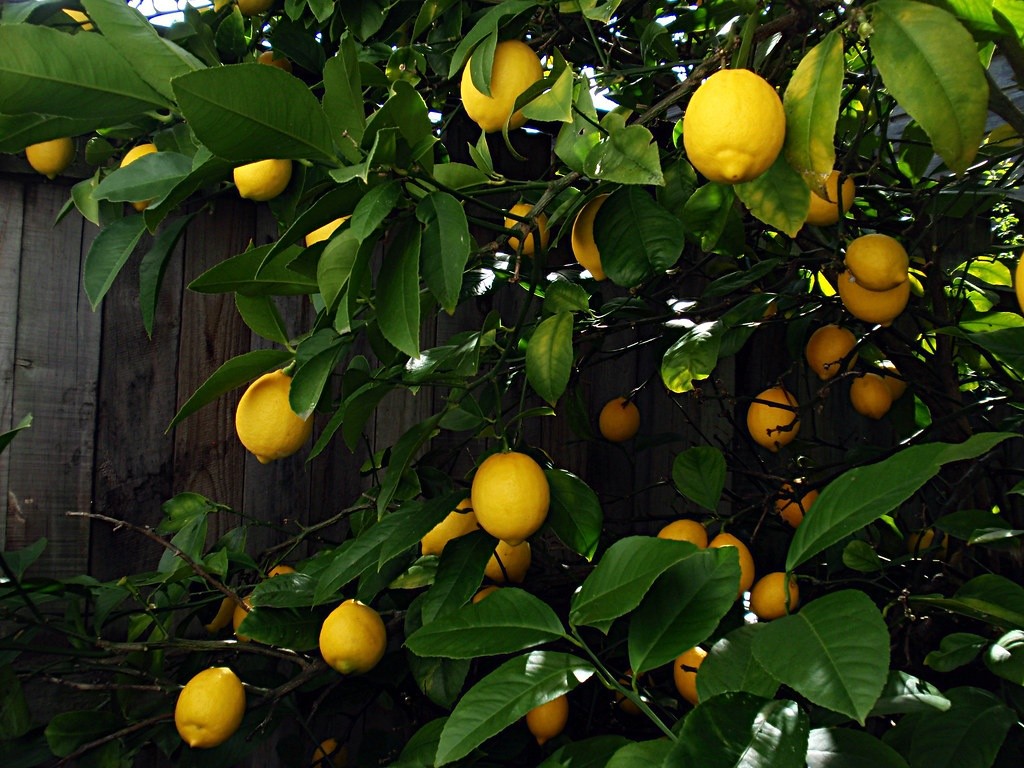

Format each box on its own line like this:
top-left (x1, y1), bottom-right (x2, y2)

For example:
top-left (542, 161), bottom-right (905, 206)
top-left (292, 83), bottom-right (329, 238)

top-left (27, 6), bottom-right (1024, 764)
